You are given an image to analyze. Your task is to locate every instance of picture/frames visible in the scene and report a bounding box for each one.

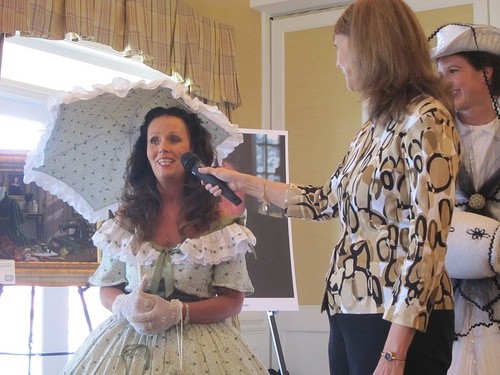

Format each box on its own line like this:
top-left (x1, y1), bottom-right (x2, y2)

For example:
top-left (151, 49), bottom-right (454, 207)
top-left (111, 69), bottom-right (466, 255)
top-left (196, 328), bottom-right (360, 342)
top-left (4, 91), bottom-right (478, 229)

top-left (0, 153), bottom-right (103, 287)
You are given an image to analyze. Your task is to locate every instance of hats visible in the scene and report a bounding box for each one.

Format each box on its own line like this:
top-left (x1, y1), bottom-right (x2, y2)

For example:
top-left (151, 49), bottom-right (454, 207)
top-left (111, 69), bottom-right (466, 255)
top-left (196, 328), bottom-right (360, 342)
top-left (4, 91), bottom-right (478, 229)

top-left (427, 21), bottom-right (500, 57)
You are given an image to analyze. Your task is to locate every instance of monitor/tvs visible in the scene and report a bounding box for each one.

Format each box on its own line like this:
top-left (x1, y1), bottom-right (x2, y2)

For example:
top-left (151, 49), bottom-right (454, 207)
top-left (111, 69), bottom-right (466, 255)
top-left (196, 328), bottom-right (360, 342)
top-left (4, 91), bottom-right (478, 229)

top-left (21, 215), bottom-right (39, 242)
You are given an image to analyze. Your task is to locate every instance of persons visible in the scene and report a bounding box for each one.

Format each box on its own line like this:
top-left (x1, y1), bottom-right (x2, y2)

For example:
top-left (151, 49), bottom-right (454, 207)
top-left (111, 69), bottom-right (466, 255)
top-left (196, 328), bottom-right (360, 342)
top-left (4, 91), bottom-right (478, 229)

top-left (430, 22), bottom-right (500, 375)
top-left (58, 107), bottom-right (271, 375)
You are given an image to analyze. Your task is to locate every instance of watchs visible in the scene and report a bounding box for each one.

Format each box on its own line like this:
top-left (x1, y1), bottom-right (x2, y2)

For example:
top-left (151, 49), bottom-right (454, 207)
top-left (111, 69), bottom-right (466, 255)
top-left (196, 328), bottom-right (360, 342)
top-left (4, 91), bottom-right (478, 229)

top-left (380, 351), bottom-right (406, 362)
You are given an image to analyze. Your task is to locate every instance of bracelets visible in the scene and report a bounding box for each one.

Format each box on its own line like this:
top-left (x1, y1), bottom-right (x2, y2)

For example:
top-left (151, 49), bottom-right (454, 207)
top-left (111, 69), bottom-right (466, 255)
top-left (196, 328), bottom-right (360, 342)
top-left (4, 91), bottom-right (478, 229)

top-left (258, 179), bottom-right (270, 205)
top-left (198, 0), bottom-right (460, 375)
top-left (182, 301), bottom-right (190, 326)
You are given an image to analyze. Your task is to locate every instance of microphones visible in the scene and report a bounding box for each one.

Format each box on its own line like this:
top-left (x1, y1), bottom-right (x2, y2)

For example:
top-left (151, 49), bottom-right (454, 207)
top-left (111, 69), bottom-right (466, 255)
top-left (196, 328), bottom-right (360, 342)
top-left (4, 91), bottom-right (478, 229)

top-left (180, 152), bottom-right (242, 206)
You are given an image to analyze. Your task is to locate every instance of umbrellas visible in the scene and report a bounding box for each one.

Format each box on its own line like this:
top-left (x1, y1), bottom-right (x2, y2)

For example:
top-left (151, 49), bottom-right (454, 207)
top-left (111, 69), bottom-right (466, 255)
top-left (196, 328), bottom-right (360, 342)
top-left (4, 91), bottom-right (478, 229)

top-left (23, 72), bottom-right (243, 292)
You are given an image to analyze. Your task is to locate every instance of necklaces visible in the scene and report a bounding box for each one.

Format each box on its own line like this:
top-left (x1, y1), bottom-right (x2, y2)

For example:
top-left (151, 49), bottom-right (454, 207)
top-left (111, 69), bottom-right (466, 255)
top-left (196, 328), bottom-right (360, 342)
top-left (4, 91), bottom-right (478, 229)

top-left (148, 229), bottom-right (195, 244)
